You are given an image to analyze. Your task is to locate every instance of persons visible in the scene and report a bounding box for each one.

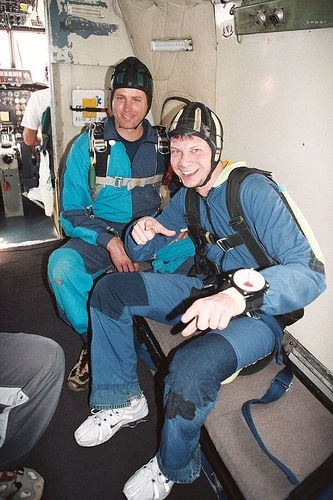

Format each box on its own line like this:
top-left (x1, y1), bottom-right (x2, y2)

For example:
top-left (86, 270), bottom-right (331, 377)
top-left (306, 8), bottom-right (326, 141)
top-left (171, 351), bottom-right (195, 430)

top-left (47, 56), bottom-right (184, 392)
top-left (73, 102), bottom-right (327, 500)
top-left (21, 65), bottom-right (52, 186)
top-left (0, 329), bottom-right (66, 499)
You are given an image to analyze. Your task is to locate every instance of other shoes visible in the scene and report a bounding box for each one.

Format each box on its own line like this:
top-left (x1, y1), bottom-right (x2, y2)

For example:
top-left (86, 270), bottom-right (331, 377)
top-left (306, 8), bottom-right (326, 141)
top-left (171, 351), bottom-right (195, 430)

top-left (0, 466), bottom-right (45, 500)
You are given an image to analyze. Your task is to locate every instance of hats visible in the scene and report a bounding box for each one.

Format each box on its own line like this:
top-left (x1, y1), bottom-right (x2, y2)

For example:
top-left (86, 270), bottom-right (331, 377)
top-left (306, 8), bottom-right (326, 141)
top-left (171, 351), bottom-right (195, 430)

top-left (111, 57), bottom-right (153, 117)
top-left (167, 102), bottom-right (223, 168)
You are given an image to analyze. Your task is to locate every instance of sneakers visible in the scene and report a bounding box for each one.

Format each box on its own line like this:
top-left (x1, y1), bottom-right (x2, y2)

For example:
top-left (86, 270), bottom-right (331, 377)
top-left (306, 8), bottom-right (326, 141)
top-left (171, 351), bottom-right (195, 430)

top-left (74, 394), bottom-right (148, 448)
top-left (66, 345), bottom-right (91, 393)
top-left (123, 455), bottom-right (174, 500)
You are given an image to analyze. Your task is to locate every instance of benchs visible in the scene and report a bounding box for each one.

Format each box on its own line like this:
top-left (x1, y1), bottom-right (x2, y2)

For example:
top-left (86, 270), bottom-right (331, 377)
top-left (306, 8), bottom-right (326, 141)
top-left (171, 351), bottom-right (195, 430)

top-left (107, 258), bottom-right (333, 500)
